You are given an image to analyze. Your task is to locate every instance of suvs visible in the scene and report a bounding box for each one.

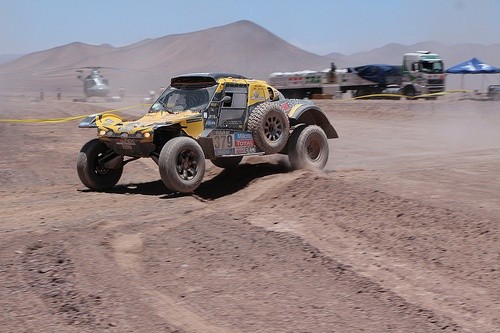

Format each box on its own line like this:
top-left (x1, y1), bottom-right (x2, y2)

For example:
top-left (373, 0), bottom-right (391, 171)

top-left (76, 72), bottom-right (339, 192)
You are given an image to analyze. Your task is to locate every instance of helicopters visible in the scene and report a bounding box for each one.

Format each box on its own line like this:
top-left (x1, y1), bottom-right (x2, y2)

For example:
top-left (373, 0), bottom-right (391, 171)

top-left (67, 66), bottom-right (121, 101)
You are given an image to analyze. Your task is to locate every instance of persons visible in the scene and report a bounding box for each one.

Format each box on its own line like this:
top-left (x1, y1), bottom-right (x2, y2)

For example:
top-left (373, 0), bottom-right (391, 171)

top-left (185, 87), bottom-right (210, 111)
top-left (329, 61), bottom-right (337, 84)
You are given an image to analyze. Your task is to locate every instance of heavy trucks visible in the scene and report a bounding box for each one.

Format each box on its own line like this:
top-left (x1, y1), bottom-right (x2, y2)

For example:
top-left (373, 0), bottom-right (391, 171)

top-left (269, 51), bottom-right (446, 100)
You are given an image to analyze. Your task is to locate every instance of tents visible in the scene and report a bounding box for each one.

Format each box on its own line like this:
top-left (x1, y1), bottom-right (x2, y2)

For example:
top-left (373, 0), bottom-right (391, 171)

top-left (444, 56), bottom-right (500, 98)
